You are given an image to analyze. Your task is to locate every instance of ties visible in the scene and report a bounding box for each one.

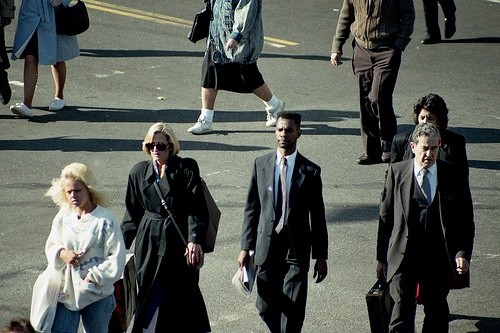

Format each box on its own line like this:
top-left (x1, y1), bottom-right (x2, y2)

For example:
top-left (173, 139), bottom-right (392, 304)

top-left (273, 158), bottom-right (288, 234)
top-left (420, 169), bottom-right (431, 204)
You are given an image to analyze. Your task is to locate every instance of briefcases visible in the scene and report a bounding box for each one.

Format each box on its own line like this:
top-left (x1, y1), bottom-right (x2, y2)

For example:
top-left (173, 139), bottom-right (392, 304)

top-left (365, 268), bottom-right (394, 333)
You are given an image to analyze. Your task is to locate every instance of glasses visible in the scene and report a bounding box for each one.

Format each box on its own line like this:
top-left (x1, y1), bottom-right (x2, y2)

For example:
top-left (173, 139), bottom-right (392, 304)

top-left (145, 141), bottom-right (170, 151)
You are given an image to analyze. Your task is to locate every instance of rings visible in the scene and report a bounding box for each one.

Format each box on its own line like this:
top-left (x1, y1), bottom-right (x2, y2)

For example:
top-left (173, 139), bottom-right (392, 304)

top-left (200, 252), bottom-right (203, 254)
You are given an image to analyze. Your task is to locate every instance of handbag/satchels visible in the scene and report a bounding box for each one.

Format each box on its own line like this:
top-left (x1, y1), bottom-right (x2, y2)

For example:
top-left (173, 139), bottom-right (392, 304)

top-left (54, 0), bottom-right (90, 36)
top-left (112, 253), bottom-right (138, 331)
top-left (181, 157), bottom-right (221, 253)
top-left (186, 251), bottom-right (204, 269)
top-left (30, 263), bottom-right (64, 333)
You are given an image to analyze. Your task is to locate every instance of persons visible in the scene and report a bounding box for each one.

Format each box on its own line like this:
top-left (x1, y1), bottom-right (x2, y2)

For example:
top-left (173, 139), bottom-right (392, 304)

top-left (389, 93), bottom-right (470, 304)
top-left (0, 0), bottom-right (16, 104)
top-left (11, 0), bottom-right (81, 118)
top-left (420, 0), bottom-right (457, 44)
top-left (118, 122), bottom-right (211, 333)
top-left (330, 0), bottom-right (416, 165)
top-left (376, 123), bottom-right (476, 333)
top-left (43, 162), bottom-right (126, 333)
top-left (237, 111), bottom-right (328, 333)
top-left (186, 0), bottom-right (286, 133)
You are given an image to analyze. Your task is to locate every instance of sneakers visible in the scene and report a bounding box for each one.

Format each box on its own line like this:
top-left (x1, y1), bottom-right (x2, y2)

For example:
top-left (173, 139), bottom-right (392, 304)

top-left (10, 102), bottom-right (32, 116)
top-left (187, 116), bottom-right (213, 133)
top-left (266, 99), bottom-right (285, 126)
top-left (49, 98), bottom-right (66, 110)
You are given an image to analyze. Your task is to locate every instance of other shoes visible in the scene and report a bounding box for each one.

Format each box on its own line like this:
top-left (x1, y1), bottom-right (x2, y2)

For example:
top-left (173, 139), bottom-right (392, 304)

top-left (356, 153), bottom-right (380, 165)
top-left (380, 151), bottom-right (392, 162)
top-left (421, 32), bottom-right (441, 43)
top-left (444, 15), bottom-right (456, 39)
top-left (0, 83), bottom-right (12, 105)
